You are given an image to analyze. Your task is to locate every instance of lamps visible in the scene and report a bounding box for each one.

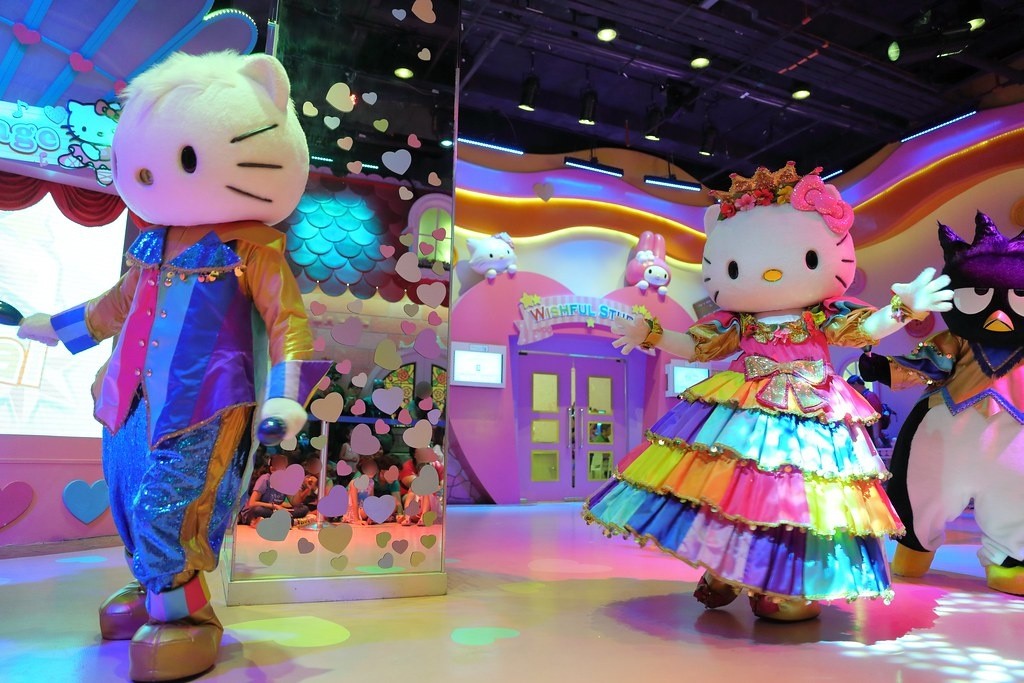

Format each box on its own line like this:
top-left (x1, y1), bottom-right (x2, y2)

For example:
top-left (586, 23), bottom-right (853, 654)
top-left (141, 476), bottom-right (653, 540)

top-left (789, 82), bottom-right (809, 100)
top-left (688, 48), bottom-right (710, 69)
top-left (564, 155), bottom-right (623, 178)
top-left (644, 173), bottom-right (703, 192)
top-left (578, 91), bottom-right (599, 127)
top-left (886, 21), bottom-right (974, 62)
top-left (595, 21), bottom-right (617, 41)
top-left (518, 70), bottom-right (543, 110)
top-left (459, 132), bottom-right (526, 156)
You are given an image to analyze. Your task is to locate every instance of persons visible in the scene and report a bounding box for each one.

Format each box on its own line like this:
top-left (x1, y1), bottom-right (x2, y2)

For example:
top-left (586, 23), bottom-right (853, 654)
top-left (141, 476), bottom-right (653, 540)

top-left (240, 364), bottom-right (445, 527)
top-left (570, 402), bottom-right (614, 488)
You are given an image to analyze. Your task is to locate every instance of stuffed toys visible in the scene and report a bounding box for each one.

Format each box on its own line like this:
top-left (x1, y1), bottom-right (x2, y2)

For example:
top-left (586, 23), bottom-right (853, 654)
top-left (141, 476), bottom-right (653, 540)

top-left (19, 52), bottom-right (336, 683)
top-left (625, 231), bottom-right (671, 297)
top-left (859, 210), bottom-right (1024, 595)
top-left (584, 160), bottom-right (954, 623)
top-left (465, 232), bottom-right (517, 279)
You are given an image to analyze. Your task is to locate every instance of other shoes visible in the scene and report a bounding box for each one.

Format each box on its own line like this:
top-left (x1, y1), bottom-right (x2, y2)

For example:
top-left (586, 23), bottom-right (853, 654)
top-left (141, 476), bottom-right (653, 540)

top-left (418, 519), bottom-right (425, 526)
top-left (250, 516), bottom-right (265, 527)
top-left (296, 518), bottom-right (312, 526)
top-left (402, 516), bottom-right (411, 525)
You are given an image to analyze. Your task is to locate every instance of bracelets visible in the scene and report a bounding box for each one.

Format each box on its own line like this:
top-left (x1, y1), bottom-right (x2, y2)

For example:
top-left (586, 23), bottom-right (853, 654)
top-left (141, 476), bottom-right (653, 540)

top-left (640, 317), bottom-right (663, 352)
top-left (271, 502), bottom-right (274, 509)
top-left (891, 293), bottom-right (929, 322)
top-left (314, 488), bottom-right (318, 494)
